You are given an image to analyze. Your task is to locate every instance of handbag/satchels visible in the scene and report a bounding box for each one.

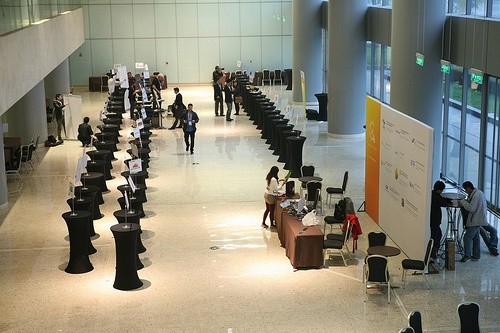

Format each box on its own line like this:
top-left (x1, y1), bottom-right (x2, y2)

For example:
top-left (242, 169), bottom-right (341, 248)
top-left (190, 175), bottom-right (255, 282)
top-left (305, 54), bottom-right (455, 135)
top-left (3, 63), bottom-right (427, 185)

top-left (48, 135), bottom-right (57, 143)
top-left (301, 209), bottom-right (320, 226)
top-left (184, 120), bottom-right (194, 133)
top-left (45, 139), bottom-right (63, 147)
top-left (235, 96), bottom-right (242, 103)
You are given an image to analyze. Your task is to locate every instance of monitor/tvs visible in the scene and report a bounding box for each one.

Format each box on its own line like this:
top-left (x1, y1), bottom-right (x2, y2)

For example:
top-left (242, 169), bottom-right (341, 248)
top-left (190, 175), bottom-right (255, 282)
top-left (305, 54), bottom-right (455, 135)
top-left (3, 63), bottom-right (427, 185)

top-left (297, 196), bottom-right (306, 215)
top-left (286, 181), bottom-right (294, 197)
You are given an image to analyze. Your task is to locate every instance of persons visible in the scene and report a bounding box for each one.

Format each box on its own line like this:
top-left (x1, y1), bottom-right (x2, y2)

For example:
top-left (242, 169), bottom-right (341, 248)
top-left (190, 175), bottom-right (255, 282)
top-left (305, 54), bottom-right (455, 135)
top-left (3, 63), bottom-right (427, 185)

top-left (127, 72), bottom-right (135, 88)
top-left (77, 117), bottom-right (94, 147)
top-left (168, 88), bottom-right (183, 130)
top-left (53, 93), bottom-right (69, 140)
top-left (232, 79), bottom-right (240, 115)
top-left (460, 206), bottom-right (499, 257)
top-left (107, 74), bottom-right (126, 95)
top-left (128, 79), bottom-right (140, 119)
top-left (212, 66), bottom-right (223, 101)
top-left (180, 103), bottom-right (199, 154)
top-left (430, 180), bottom-right (454, 259)
top-left (457, 181), bottom-right (488, 262)
top-left (151, 74), bottom-right (164, 108)
top-left (213, 76), bottom-right (225, 116)
top-left (261, 166), bottom-right (284, 229)
top-left (224, 79), bottom-right (236, 122)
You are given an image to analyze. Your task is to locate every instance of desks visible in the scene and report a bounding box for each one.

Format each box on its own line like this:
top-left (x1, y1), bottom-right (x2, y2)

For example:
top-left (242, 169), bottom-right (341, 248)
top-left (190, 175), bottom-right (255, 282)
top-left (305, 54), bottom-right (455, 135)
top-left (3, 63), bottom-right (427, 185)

top-left (443, 193), bottom-right (466, 254)
top-left (274, 193), bottom-right (324, 270)
top-left (234, 69), bottom-right (328, 182)
top-left (61, 75), bottom-right (155, 291)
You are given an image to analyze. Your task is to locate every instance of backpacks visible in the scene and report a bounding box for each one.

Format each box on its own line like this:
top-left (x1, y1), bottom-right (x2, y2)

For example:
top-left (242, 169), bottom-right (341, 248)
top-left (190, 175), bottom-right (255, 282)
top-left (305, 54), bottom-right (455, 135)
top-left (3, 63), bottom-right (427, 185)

top-left (334, 200), bottom-right (346, 220)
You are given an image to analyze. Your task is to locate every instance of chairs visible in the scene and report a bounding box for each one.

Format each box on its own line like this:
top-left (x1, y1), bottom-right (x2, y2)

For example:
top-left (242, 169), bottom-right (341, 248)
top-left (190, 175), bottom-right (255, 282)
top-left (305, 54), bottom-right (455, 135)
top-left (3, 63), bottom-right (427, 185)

top-left (8, 183), bottom-right (23, 197)
top-left (300, 165), bottom-right (434, 303)
top-left (47, 106), bottom-right (56, 128)
top-left (400, 310), bottom-right (425, 333)
top-left (7, 135), bottom-right (41, 180)
top-left (457, 302), bottom-right (481, 333)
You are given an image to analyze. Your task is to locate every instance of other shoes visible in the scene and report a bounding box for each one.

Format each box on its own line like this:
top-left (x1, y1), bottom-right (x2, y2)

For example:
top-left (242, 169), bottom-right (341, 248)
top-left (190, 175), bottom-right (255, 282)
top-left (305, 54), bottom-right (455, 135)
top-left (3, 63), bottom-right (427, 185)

top-left (226, 119), bottom-right (233, 121)
top-left (191, 151), bottom-right (193, 153)
top-left (186, 146), bottom-right (189, 151)
top-left (471, 256), bottom-right (480, 260)
top-left (176, 126), bottom-right (182, 128)
top-left (271, 225), bottom-right (277, 229)
top-left (168, 127), bottom-right (175, 129)
top-left (216, 114), bottom-right (218, 116)
top-left (491, 247), bottom-right (499, 256)
top-left (234, 113), bottom-right (239, 115)
top-left (220, 115), bottom-right (225, 116)
top-left (261, 223), bottom-right (268, 228)
top-left (460, 255), bottom-right (470, 263)
